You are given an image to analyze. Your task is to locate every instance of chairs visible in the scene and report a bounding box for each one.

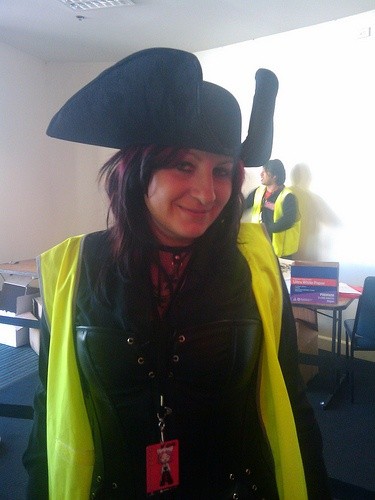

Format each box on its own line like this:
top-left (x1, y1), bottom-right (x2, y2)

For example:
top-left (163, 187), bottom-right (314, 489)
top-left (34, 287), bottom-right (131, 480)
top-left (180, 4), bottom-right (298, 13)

top-left (344, 276), bottom-right (375, 385)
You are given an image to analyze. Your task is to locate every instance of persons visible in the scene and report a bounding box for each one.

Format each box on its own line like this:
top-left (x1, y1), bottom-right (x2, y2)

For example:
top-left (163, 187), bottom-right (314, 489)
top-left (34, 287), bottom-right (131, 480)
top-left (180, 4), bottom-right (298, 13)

top-left (21, 48), bottom-right (328, 500)
top-left (244, 160), bottom-right (302, 260)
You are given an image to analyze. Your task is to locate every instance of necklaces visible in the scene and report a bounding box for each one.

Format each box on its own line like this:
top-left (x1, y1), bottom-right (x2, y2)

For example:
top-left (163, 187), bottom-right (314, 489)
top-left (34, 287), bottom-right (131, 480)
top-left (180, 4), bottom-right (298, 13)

top-left (159, 244), bottom-right (193, 267)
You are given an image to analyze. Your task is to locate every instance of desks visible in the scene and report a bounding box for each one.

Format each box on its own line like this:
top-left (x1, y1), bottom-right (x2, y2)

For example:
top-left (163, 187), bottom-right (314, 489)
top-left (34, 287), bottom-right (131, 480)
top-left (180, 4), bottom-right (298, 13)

top-left (290, 293), bottom-right (352, 409)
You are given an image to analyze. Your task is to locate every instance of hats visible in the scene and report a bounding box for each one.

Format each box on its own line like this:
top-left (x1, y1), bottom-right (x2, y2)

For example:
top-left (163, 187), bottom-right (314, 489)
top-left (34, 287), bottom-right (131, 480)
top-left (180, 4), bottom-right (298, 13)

top-left (46, 47), bottom-right (279, 167)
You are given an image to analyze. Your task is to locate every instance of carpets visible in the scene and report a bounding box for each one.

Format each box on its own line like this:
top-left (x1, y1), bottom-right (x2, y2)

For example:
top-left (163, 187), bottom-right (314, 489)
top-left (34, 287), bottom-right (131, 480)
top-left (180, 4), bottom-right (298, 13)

top-left (0, 343), bottom-right (39, 388)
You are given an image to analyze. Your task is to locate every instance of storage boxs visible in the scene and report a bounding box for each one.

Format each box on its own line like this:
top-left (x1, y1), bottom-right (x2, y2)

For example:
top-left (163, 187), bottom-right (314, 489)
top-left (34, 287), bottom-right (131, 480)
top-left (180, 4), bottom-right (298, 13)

top-left (290, 262), bottom-right (339, 305)
top-left (0, 281), bottom-right (42, 356)
